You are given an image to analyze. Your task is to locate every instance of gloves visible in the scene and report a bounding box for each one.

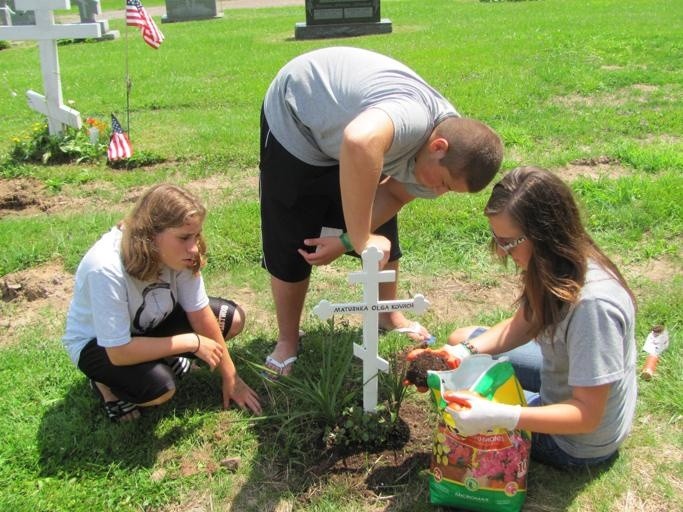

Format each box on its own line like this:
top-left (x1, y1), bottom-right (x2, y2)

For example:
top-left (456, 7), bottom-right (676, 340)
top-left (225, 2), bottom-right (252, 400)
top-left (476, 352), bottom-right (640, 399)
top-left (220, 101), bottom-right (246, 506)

top-left (402, 343), bottom-right (473, 393)
top-left (442, 390), bottom-right (521, 437)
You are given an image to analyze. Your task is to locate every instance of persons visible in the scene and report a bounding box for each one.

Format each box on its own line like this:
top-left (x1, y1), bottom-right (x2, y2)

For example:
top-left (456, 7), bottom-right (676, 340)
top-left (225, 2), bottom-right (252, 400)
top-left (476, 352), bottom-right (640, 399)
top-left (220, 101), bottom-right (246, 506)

top-left (66, 183), bottom-right (263, 425)
top-left (253, 45), bottom-right (502, 381)
top-left (404, 166), bottom-right (638, 472)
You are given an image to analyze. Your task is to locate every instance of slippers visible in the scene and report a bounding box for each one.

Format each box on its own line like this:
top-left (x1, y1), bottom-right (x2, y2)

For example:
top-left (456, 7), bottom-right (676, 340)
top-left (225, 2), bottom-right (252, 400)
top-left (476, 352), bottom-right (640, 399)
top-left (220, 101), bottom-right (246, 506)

top-left (89, 378), bottom-right (142, 423)
top-left (390, 321), bottom-right (437, 345)
top-left (170, 357), bottom-right (191, 380)
top-left (264, 355), bottom-right (297, 376)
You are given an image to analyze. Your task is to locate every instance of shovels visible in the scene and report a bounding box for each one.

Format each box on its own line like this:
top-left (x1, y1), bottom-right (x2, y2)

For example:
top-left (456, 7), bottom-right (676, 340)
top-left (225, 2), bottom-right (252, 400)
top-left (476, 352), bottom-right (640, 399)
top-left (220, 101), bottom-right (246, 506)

top-left (640, 326), bottom-right (670, 383)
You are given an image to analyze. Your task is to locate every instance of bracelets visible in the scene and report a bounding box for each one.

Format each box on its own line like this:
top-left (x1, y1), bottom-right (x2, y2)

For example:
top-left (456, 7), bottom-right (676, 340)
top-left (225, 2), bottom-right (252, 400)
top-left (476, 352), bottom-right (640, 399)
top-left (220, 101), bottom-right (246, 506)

top-left (462, 339), bottom-right (479, 354)
top-left (191, 332), bottom-right (200, 354)
top-left (339, 233), bottom-right (354, 252)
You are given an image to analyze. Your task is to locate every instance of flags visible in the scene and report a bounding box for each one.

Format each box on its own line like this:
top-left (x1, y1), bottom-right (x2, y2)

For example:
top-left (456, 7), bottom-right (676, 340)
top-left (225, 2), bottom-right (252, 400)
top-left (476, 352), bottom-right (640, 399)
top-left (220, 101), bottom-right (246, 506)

top-left (123, 0), bottom-right (166, 52)
top-left (106, 111), bottom-right (133, 161)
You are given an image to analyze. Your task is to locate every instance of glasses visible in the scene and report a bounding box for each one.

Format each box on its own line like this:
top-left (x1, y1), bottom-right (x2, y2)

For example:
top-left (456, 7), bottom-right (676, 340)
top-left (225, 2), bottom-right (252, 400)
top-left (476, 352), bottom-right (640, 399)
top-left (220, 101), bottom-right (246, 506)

top-left (492, 231), bottom-right (527, 253)
